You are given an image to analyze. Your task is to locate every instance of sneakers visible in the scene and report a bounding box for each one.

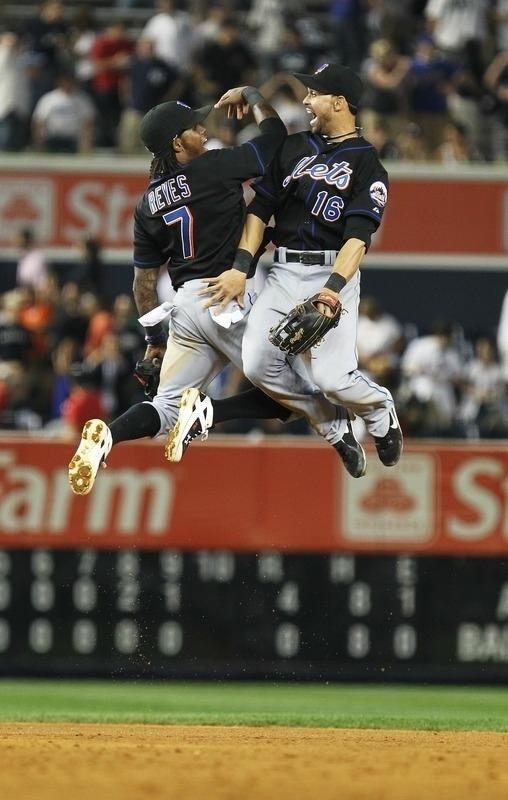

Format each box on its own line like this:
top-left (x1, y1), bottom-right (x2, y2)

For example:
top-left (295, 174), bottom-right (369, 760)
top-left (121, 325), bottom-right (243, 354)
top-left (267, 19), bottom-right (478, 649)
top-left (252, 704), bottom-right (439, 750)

top-left (366, 385), bottom-right (405, 467)
top-left (68, 418), bottom-right (114, 496)
top-left (165, 387), bottom-right (216, 462)
top-left (324, 405), bottom-right (367, 479)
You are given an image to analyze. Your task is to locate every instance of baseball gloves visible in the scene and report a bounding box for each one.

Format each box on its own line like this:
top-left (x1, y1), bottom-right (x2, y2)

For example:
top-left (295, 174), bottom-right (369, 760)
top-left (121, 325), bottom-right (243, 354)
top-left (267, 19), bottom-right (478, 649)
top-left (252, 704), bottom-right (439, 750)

top-left (135, 358), bottom-right (161, 399)
top-left (267, 293), bottom-right (343, 355)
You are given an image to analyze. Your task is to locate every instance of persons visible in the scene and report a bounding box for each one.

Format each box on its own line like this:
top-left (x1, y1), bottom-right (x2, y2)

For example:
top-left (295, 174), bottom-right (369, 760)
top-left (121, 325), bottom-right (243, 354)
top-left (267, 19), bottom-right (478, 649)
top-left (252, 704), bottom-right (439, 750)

top-left (193, 52), bottom-right (406, 477)
top-left (0, 0), bottom-right (508, 163)
top-left (1, 228), bottom-right (508, 438)
top-left (63, 83), bottom-right (289, 498)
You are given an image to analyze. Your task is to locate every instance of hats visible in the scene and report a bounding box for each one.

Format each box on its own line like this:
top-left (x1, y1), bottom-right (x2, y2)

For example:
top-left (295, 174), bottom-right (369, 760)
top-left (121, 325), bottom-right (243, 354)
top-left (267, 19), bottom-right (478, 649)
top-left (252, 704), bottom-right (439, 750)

top-left (140, 99), bottom-right (213, 155)
top-left (292, 62), bottom-right (364, 110)
